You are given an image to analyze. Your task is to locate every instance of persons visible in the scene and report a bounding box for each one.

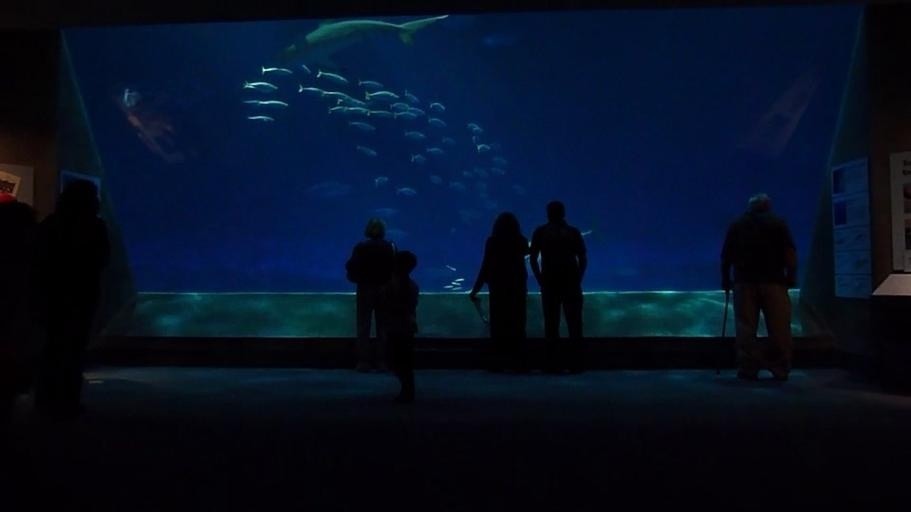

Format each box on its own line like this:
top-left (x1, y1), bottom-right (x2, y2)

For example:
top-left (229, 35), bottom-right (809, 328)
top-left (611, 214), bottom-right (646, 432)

top-left (721, 193), bottom-right (797, 381)
top-left (347, 219), bottom-right (396, 372)
top-left (468, 212), bottom-right (530, 374)
top-left (529, 201), bottom-right (586, 375)
top-left (374, 252), bottom-right (417, 403)
top-left (1, 175), bottom-right (141, 458)
